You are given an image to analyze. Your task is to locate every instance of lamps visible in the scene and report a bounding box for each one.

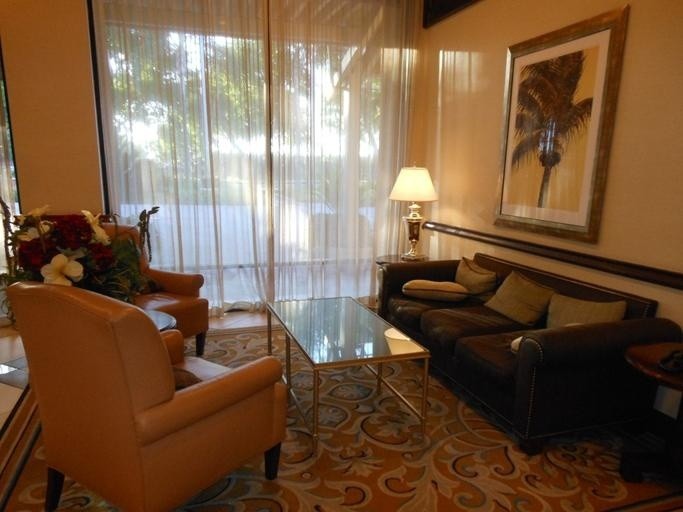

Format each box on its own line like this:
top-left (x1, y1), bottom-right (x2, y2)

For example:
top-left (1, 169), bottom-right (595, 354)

top-left (383, 328), bottom-right (425, 356)
top-left (389, 161), bottom-right (438, 261)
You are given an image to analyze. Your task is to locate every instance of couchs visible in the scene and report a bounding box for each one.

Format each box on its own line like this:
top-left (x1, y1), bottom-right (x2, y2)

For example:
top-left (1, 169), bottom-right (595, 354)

top-left (377, 253), bottom-right (683, 457)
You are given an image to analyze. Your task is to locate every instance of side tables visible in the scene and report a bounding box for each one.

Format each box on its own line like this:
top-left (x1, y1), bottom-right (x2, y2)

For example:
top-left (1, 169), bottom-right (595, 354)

top-left (373, 255), bottom-right (430, 266)
top-left (615, 341), bottom-right (683, 483)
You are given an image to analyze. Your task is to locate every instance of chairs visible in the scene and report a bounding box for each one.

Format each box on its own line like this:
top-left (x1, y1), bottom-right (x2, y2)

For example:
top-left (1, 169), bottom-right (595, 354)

top-left (6, 280), bottom-right (289, 512)
top-left (97, 220), bottom-right (210, 357)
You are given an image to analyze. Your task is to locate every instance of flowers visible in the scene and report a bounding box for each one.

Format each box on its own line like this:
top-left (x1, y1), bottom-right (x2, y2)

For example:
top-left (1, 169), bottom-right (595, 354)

top-left (5, 203), bottom-right (147, 303)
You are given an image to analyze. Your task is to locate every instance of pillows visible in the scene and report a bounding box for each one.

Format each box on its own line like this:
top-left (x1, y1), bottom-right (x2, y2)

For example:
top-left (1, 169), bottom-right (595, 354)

top-left (402, 256), bottom-right (629, 329)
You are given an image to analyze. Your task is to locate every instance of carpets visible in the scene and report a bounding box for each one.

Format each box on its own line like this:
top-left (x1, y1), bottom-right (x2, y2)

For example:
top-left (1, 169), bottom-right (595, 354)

top-left (0, 306), bottom-right (683, 512)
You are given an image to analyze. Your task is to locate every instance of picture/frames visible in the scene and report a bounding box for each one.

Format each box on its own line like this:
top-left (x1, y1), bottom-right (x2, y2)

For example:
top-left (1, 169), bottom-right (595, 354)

top-left (490, 3), bottom-right (630, 243)
